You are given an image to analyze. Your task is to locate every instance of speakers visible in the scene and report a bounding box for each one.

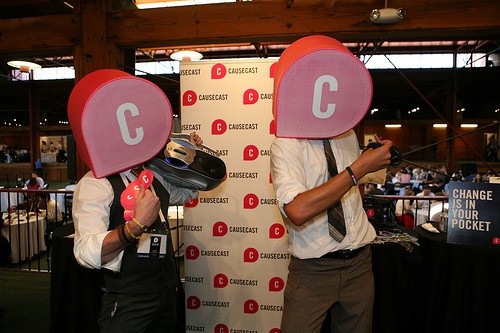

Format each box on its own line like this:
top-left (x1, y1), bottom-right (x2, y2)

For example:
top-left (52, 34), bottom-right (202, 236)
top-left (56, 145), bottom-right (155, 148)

top-left (144, 133), bottom-right (226, 191)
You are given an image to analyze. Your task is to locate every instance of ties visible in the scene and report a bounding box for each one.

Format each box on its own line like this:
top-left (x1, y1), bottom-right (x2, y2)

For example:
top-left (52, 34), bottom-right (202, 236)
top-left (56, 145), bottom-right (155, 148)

top-left (322, 138), bottom-right (347, 243)
top-left (130, 167), bottom-right (145, 178)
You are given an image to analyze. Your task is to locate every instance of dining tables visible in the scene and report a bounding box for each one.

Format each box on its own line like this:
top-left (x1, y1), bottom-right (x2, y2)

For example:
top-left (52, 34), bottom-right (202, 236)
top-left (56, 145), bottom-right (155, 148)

top-left (3, 170), bottom-right (499, 268)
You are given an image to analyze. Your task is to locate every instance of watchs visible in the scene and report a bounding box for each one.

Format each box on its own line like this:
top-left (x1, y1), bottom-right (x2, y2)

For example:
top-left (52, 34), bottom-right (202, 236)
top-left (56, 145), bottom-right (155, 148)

top-left (131, 218), bottom-right (151, 234)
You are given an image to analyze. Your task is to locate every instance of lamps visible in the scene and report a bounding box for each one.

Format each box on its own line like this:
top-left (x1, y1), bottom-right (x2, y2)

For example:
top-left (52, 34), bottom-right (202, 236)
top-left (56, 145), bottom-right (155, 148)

top-left (168, 49), bottom-right (204, 62)
top-left (6, 56), bottom-right (42, 73)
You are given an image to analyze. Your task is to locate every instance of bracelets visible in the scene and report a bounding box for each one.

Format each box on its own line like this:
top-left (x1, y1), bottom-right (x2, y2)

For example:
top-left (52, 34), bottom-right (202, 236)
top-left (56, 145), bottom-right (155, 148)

top-left (117, 221), bottom-right (141, 247)
top-left (345, 166), bottom-right (358, 186)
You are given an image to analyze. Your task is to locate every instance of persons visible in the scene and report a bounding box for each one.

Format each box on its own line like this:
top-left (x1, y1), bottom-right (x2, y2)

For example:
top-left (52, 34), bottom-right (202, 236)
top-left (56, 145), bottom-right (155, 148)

top-left (365, 134), bottom-right (500, 234)
top-left (67, 91), bottom-right (205, 333)
top-left (270, 74), bottom-right (393, 333)
top-left (0, 143), bottom-right (30, 164)
top-left (24, 173), bottom-right (47, 206)
top-left (41, 139), bottom-right (66, 163)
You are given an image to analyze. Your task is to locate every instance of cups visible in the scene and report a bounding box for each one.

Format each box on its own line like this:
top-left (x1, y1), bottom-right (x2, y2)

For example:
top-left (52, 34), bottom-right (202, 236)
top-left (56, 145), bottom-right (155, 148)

top-left (440, 212), bottom-right (448, 232)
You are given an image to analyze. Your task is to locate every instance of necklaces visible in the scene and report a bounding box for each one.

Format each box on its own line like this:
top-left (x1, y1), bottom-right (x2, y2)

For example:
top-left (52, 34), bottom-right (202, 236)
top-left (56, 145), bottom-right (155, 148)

top-left (119, 164), bottom-right (168, 257)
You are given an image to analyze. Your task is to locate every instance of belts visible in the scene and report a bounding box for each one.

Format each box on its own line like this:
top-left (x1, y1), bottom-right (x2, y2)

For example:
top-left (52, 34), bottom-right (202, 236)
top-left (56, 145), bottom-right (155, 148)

top-left (321, 247), bottom-right (364, 260)
